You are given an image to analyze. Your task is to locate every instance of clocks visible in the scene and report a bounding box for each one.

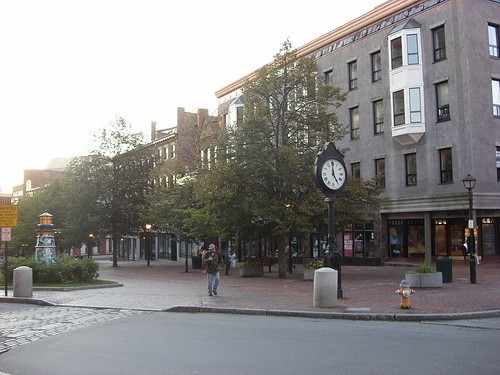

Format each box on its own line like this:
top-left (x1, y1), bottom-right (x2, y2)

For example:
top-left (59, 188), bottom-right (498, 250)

top-left (321, 158), bottom-right (345, 189)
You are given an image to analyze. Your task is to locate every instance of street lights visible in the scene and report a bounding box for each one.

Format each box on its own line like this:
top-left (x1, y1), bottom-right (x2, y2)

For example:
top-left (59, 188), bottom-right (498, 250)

top-left (462, 174), bottom-right (477, 285)
top-left (146, 223), bottom-right (152, 266)
top-left (89, 233), bottom-right (94, 260)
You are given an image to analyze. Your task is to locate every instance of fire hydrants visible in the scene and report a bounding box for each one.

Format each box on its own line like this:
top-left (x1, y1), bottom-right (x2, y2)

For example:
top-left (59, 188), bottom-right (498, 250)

top-left (395, 279), bottom-right (415, 310)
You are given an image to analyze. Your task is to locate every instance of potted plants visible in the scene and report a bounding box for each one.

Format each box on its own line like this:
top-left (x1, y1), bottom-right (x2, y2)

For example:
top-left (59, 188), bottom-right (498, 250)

top-left (240, 261), bottom-right (263, 277)
top-left (303, 259), bottom-right (323, 280)
top-left (405, 262), bottom-right (444, 288)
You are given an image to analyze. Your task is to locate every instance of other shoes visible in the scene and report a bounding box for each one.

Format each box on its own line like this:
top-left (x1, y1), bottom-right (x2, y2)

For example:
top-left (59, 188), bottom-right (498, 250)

top-left (213, 291), bottom-right (217, 294)
top-left (209, 293), bottom-right (212, 296)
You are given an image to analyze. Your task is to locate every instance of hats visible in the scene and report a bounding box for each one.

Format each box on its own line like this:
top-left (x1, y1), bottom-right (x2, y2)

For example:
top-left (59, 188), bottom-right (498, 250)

top-left (208, 244), bottom-right (215, 249)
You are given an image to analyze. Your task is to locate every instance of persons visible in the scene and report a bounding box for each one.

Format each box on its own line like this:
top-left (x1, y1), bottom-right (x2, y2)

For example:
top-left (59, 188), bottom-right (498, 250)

top-left (199, 247), bottom-right (208, 273)
top-left (203, 244), bottom-right (225, 296)
